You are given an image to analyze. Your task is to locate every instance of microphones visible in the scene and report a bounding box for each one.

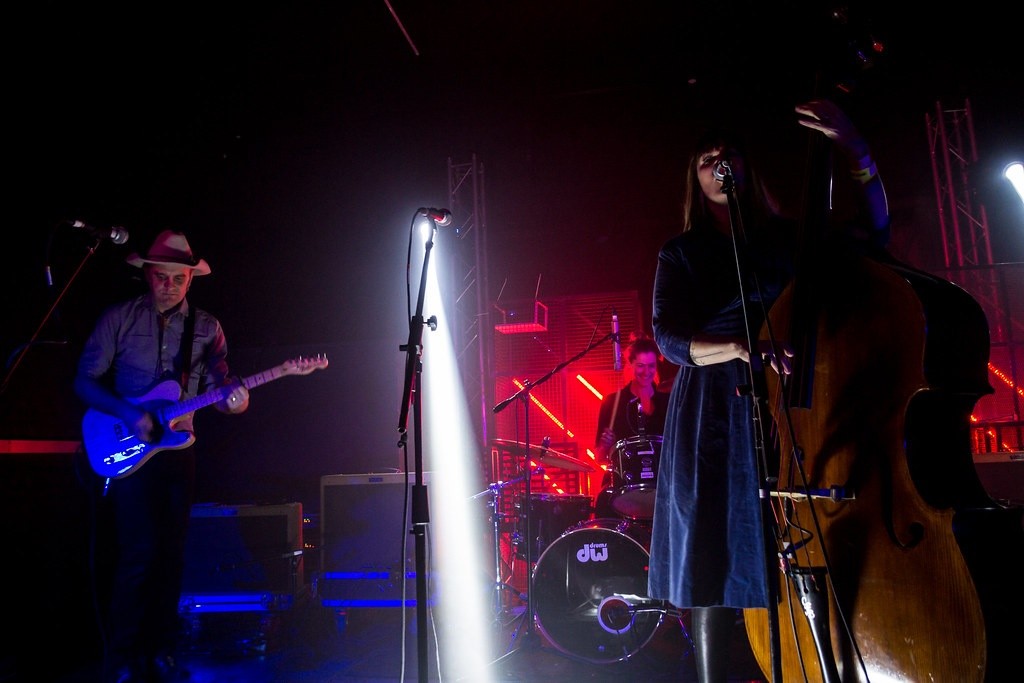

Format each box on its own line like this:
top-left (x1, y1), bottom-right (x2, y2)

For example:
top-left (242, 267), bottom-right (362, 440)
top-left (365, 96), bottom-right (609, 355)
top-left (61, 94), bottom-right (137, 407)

top-left (418, 207), bottom-right (452, 227)
top-left (612, 304), bottom-right (622, 371)
top-left (72, 220), bottom-right (129, 244)
top-left (608, 606), bottom-right (620, 625)
top-left (712, 160), bottom-right (731, 182)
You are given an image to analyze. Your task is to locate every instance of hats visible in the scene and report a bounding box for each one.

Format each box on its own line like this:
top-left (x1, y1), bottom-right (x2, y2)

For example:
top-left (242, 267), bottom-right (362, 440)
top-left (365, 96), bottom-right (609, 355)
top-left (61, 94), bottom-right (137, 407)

top-left (124, 229), bottom-right (212, 277)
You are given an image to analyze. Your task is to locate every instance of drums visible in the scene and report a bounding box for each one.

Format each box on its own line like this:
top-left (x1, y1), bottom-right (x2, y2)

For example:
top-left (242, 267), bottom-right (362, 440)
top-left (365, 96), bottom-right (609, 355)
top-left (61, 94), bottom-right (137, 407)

top-left (603, 433), bottom-right (666, 525)
top-left (530, 518), bottom-right (685, 666)
top-left (509, 490), bottom-right (596, 564)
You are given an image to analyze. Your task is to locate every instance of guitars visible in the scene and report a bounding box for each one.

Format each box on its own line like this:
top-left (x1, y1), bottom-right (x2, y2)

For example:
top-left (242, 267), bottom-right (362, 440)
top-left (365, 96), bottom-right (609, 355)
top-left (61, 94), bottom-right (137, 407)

top-left (81, 348), bottom-right (329, 481)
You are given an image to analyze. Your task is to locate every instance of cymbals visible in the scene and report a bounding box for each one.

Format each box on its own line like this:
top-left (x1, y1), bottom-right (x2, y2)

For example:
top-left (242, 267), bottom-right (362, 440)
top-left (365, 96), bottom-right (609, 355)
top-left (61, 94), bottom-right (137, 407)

top-left (489, 436), bottom-right (592, 473)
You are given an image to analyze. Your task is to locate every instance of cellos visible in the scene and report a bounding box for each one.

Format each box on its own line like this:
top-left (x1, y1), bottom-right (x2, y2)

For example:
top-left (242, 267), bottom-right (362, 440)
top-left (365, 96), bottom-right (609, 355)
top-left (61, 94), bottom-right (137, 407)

top-left (739, 4), bottom-right (1023, 683)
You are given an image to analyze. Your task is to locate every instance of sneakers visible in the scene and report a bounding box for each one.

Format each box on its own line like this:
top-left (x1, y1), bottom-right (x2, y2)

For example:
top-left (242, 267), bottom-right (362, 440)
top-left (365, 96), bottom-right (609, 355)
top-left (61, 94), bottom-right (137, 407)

top-left (99, 656), bottom-right (171, 683)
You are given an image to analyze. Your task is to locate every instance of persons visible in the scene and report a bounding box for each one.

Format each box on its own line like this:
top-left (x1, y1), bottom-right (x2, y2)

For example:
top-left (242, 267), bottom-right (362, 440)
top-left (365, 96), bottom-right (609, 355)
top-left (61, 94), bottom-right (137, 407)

top-left (595, 332), bottom-right (672, 536)
top-left (650, 94), bottom-right (893, 683)
top-left (75, 230), bottom-right (251, 683)
